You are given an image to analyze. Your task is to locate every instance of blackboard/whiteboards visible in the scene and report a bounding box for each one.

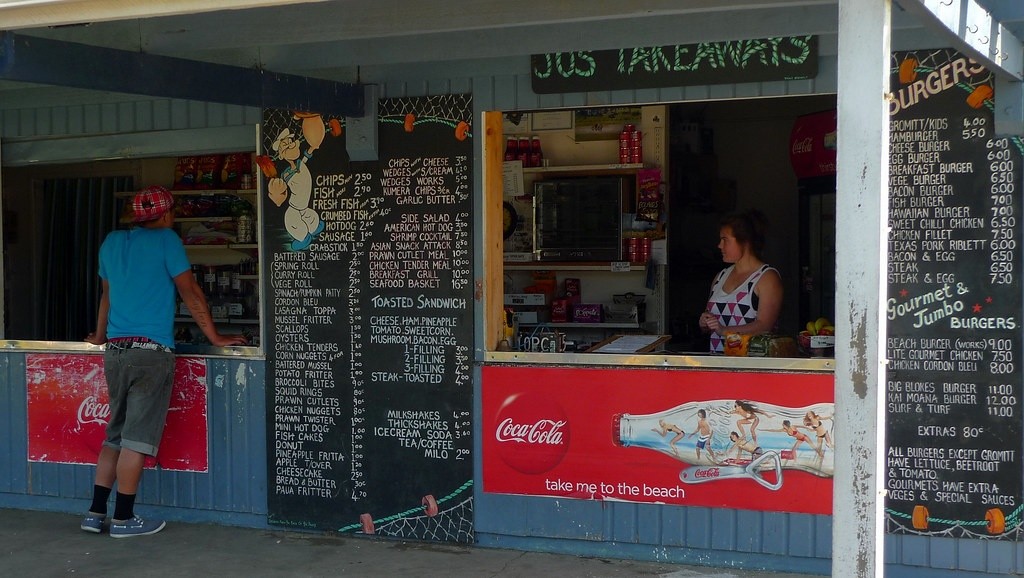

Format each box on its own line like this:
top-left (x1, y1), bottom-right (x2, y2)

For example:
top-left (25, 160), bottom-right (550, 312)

top-left (884, 46), bottom-right (1024, 541)
top-left (260, 93), bottom-right (475, 546)
top-left (530, 36), bottom-right (820, 94)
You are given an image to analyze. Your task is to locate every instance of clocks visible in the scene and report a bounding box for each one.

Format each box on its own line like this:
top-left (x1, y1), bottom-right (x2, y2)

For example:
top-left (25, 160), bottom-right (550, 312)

top-left (502, 202), bottom-right (518, 239)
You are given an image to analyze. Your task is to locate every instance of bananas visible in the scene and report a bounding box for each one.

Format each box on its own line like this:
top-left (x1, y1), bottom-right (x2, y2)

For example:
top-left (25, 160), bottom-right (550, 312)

top-left (806, 318), bottom-right (835, 336)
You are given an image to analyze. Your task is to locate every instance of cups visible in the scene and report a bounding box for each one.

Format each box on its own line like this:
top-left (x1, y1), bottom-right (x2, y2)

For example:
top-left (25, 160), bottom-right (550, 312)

top-left (237, 215), bottom-right (256, 244)
top-left (619, 125), bottom-right (642, 164)
top-left (622, 237), bottom-right (650, 264)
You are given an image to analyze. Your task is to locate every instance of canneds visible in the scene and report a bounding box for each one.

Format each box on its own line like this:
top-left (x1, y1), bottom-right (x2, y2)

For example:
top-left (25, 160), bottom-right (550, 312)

top-left (619, 124), bottom-right (640, 164)
top-left (622, 237), bottom-right (652, 264)
top-left (240, 173), bottom-right (253, 189)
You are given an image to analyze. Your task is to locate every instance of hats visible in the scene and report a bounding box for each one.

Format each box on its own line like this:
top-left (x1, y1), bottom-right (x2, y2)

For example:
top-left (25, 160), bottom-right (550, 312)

top-left (131, 185), bottom-right (175, 223)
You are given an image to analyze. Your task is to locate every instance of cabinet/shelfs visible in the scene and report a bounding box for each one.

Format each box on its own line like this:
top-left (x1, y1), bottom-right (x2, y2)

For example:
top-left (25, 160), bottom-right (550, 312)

top-left (113, 185), bottom-right (260, 326)
top-left (499, 163), bottom-right (658, 332)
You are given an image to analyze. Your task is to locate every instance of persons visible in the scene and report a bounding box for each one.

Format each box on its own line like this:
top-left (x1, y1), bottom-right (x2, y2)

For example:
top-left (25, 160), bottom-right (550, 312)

top-left (698, 214), bottom-right (783, 352)
top-left (81, 185), bottom-right (249, 538)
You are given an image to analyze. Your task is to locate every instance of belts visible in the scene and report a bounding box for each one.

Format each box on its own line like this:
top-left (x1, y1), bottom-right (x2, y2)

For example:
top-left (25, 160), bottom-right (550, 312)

top-left (109, 343), bottom-right (132, 350)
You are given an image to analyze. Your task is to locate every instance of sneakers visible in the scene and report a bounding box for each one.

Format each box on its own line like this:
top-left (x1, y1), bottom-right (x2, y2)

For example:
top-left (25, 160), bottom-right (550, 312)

top-left (80, 510), bottom-right (106, 532)
top-left (110, 513), bottom-right (166, 538)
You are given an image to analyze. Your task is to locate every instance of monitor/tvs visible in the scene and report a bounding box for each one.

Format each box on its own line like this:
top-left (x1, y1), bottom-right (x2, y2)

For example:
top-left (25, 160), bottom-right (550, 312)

top-left (535, 177), bottom-right (623, 261)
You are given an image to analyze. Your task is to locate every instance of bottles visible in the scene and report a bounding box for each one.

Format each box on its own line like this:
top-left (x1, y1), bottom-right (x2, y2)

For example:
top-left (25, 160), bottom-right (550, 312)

top-left (504, 136), bottom-right (543, 168)
top-left (175, 264), bottom-right (246, 319)
